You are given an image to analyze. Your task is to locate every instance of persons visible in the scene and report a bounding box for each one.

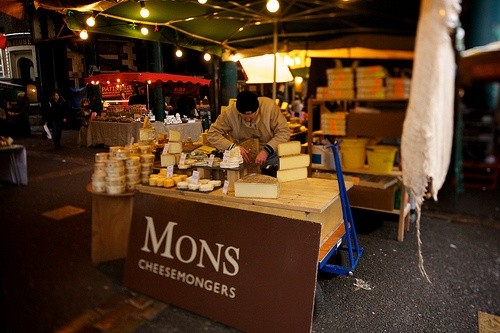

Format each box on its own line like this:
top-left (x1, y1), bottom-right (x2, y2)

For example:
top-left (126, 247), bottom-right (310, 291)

top-left (129, 86), bottom-right (148, 109)
top-left (164, 94), bottom-right (195, 118)
top-left (0, 108), bottom-right (13, 139)
top-left (41, 92), bottom-right (67, 152)
top-left (82, 84), bottom-right (102, 129)
top-left (206, 90), bottom-right (291, 166)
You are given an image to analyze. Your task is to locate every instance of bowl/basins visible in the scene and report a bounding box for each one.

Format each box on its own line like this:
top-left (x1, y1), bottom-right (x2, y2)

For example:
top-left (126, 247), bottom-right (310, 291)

top-left (92, 142), bottom-right (156, 192)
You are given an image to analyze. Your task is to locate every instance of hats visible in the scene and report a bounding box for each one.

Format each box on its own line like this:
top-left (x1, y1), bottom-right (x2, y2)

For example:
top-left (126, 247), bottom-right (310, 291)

top-left (236, 90), bottom-right (259, 115)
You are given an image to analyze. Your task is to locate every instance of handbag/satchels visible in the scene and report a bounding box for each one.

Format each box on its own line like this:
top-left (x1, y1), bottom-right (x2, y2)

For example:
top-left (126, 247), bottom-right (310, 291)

top-left (40, 131), bottom-right (50, 145)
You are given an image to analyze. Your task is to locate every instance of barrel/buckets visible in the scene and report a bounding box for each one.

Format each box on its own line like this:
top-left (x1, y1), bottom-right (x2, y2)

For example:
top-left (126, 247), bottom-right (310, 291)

top-left (366, 145), bottom-right (398, 172)
top-left (311, 142), bottom-right (336, 172)
top-left (339, 138), bottom-right (369, 168)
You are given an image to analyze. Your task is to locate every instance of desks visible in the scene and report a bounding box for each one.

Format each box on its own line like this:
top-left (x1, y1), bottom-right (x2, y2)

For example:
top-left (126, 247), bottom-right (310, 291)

top-left (89, 121), bottom-right (137, 146)
top-left (85, 183), bottom-right (134, 270)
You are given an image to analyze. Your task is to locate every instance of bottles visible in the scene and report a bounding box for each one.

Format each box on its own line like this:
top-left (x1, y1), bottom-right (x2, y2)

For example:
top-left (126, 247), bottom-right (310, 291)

top-left (395, 183), bottom-right (410, 210)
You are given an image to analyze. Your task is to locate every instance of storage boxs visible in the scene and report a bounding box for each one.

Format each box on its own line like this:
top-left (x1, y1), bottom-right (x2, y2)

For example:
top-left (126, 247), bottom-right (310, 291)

top-left (320, 113), bottom-right (347, 135)
top-left (316, 67), bottom-right (411, 100)
top-left (38, 204), bottom-right (86, 248)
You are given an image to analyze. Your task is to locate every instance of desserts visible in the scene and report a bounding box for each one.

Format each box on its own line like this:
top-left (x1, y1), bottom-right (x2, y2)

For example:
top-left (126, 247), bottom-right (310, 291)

top-left (149, 167), bottom-right (222, 191)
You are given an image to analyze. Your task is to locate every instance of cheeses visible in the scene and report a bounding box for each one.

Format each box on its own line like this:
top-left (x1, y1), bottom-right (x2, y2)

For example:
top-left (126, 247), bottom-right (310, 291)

top-left (160, 130), bottom-right (310, 199)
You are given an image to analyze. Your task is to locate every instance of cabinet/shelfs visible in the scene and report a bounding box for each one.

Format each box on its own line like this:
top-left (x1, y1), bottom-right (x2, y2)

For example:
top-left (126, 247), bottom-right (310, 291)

top-left (307, 98), bottom-right (414, 244)
